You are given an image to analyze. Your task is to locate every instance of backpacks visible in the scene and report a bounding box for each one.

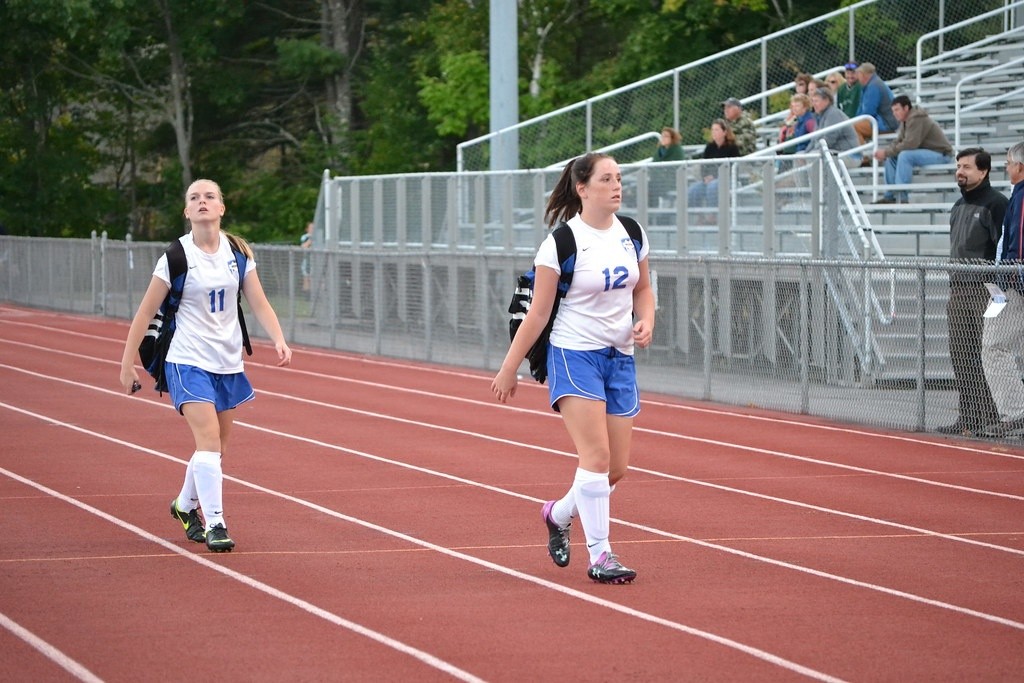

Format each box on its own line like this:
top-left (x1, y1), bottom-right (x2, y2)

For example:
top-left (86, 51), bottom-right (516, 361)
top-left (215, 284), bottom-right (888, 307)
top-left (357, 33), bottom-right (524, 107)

top-left (508, 213), bottom-right (643, 385)
top-left (138, 238), bottom-right (253, 393)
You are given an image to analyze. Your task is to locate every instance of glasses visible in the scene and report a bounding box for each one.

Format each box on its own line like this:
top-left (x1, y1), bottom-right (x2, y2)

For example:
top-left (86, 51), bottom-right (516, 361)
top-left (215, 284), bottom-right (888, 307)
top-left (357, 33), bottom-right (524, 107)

top-left (845, 64), bottom-right (856, 71)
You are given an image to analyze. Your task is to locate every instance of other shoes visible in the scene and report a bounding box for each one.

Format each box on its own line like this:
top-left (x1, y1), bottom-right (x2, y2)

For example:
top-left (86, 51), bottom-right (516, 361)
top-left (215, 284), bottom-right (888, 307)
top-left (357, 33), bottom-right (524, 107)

top-left (984, 414), bottom-right (1024, 437)
top-left (871, 196), bottom-right (909, 203)
top-left (937, 416), bottom-right (978, 434)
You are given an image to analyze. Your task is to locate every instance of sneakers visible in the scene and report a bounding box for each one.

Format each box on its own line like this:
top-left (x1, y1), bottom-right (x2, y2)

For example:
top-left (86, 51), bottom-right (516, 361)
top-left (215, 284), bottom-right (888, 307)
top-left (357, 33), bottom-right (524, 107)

top-left (203, 523), bottom-right (235, 552)
top-left (588, 547), bottom-right (637, 585)
top-left (170, 498), bottom-right (206, 543)
top-left (541, 500), bottom-right (571, 568)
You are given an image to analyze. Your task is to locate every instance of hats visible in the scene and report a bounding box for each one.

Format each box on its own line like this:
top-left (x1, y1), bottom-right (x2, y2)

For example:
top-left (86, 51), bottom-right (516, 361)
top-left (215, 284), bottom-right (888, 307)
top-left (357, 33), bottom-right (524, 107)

top-left (720, 98), bottom-right (741, 108)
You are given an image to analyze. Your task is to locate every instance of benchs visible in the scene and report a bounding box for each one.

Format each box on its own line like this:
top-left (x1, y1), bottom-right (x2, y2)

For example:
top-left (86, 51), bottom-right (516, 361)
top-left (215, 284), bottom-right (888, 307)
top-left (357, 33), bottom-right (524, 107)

top-left (458, 27), bottom-right (1023, 258)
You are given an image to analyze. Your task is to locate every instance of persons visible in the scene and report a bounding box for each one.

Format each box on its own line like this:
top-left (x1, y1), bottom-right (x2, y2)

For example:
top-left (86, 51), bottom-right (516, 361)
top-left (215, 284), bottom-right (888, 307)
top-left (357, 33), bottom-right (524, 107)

top-left (491, 153), bottom-right (656, 584)
top-left (773, 60), bottom-right (902, 210)
top-left (631, 126), bottom-right (687, 224)
top-left (937, 142), bottom-right (1024, 438)
top-left (687, 96), bottom-right (756, 209)
top-left (120, 179), bottom-right (292, 552)
top-left (872, 95), bottom-right (955, 204)
top-left (300, 222), bottom-right (315, 300)
